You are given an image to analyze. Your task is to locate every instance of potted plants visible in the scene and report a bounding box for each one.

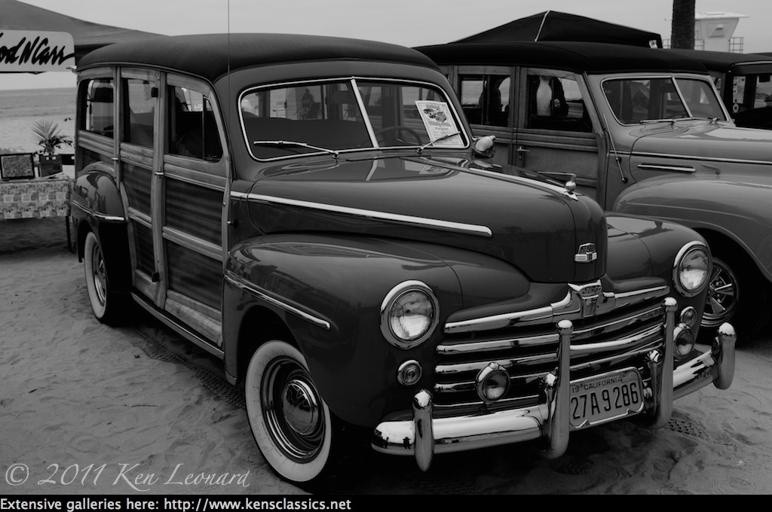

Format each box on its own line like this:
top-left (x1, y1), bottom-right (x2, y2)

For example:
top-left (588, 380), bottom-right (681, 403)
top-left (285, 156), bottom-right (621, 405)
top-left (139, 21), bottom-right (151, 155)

top-left (28, 116), bottom-right (72, 178)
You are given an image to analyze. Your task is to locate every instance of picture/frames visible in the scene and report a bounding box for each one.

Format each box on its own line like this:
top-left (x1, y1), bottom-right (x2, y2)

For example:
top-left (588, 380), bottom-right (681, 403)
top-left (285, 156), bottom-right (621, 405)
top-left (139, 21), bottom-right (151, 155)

top-left (0, 152), bottom-right (34, 179)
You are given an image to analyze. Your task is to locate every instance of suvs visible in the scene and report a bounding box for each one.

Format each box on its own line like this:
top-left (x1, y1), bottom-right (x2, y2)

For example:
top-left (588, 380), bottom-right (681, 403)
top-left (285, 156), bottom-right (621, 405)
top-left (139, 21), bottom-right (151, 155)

top-left (67, 33), bottom-right (736, 493)
top-left (568, 47), bottom-right (772, 131)
top-left (352, 10), bottom-right (772, 345)
